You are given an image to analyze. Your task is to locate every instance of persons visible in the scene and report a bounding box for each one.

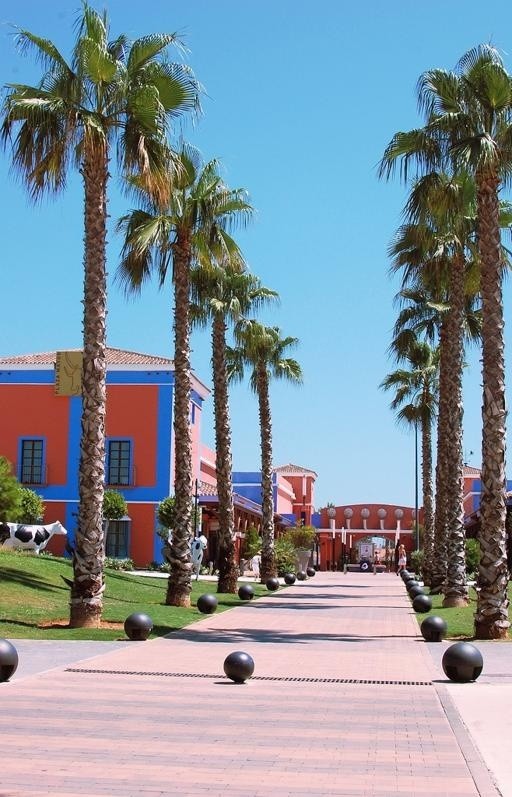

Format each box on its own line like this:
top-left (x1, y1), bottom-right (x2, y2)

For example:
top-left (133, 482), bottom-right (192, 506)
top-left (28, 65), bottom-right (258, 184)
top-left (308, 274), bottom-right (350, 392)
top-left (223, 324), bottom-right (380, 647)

top-left (394, 543), bottom-right (407, 575)
top-left (374, 550), bottom-right (380, 564)
top-left (250, 551), bottom-right (262, 581)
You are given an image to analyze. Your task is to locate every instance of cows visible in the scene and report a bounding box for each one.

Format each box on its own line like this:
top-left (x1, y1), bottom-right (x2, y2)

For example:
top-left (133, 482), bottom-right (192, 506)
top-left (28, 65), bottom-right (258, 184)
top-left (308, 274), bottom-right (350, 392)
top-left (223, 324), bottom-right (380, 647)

top-left (191, 535), bottom-right (208, 581)
top-left (0, 519), bottom-right (68, 556)
